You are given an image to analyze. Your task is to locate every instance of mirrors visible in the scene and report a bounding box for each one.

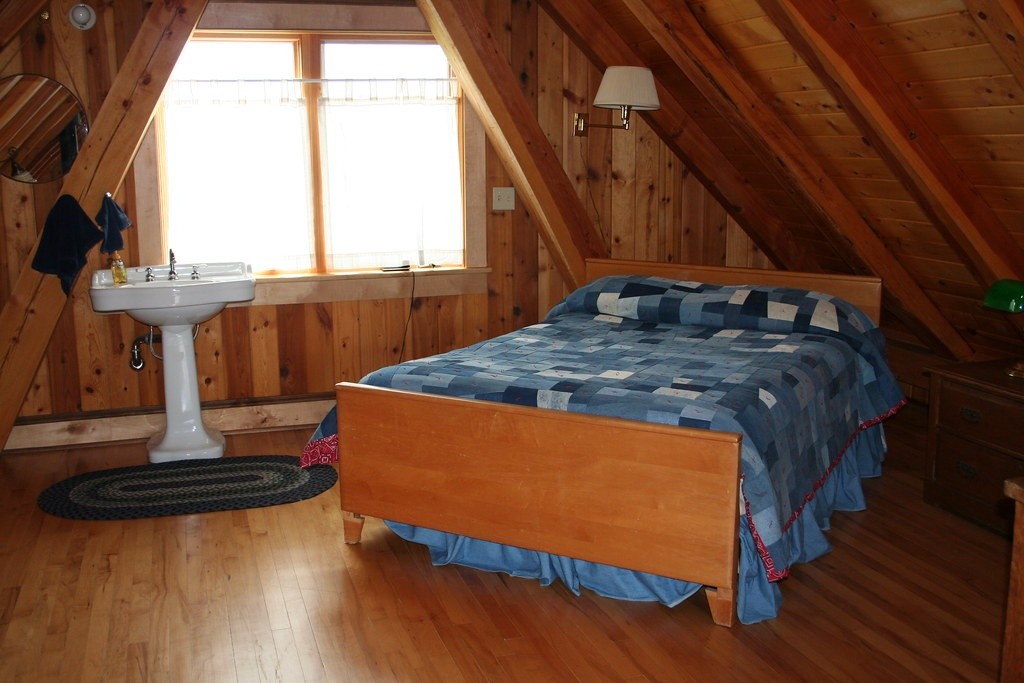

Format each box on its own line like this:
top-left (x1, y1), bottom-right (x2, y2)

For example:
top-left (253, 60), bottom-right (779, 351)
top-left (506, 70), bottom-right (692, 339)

top-left (0, 73), bottom-right (89, 183)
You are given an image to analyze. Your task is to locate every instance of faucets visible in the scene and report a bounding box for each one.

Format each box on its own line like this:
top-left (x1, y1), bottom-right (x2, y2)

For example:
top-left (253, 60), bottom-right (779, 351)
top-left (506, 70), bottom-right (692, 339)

top-left (168, 248), bottom-right (179, 280)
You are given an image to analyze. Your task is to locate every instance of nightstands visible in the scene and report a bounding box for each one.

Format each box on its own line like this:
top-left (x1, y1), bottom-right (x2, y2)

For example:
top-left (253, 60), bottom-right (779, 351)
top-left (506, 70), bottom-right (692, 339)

top-left (919, 356), bottom-right (1024, 539)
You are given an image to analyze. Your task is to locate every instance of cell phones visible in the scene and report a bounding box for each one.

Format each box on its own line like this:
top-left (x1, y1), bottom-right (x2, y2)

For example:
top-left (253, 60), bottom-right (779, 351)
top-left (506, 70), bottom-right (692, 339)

top-left (383, 265), bottom-right (410, 272)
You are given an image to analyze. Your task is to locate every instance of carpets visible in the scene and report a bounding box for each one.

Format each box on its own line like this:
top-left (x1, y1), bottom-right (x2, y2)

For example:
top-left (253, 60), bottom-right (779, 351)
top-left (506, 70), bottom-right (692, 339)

top-left (35, 455), bottom-right (338, 520)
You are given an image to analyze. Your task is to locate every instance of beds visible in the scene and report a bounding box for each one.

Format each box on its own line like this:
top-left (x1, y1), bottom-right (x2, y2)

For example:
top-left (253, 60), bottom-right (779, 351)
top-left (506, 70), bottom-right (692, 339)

top-left (300, 258), bottom-right (908, 627)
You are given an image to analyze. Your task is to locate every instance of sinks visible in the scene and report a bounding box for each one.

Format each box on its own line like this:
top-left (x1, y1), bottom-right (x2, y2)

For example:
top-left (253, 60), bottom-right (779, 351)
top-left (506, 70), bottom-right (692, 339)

top-left (89, 262), bottom-right (256, 313)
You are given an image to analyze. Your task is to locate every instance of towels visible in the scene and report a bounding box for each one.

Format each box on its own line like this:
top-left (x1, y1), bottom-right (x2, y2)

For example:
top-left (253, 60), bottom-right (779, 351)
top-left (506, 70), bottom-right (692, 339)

top-left (96, 194), bottom-right (133, 255)
top-left (32, 195), bottom-right (104, 297)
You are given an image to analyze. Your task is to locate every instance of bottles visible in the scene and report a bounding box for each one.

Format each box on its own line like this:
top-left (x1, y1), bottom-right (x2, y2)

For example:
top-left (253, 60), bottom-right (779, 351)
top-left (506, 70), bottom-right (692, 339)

top-left (112, 250), bottom-right (128, 283)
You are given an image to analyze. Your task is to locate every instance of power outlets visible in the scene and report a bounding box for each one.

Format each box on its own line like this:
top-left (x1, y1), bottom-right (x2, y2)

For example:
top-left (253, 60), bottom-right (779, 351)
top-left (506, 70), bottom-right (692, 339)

top-left (492, 187), bottom-right (515, 210)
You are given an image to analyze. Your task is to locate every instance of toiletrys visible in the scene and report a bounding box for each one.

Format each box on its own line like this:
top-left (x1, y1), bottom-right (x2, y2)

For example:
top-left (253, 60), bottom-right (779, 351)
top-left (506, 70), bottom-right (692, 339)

top-left (111, 249), bottom-right (127, 285)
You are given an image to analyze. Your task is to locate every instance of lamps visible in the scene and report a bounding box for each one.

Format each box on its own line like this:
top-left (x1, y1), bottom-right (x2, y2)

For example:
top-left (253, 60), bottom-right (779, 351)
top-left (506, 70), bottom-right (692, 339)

top-left (8, 145), bottom-right (38, 184)
top-left (982, 277), bottom-right (1024, 378)
top-left (573, 66), bottom-right (660, 136)
top-left (68, 3), bottom-right (97, 30)
